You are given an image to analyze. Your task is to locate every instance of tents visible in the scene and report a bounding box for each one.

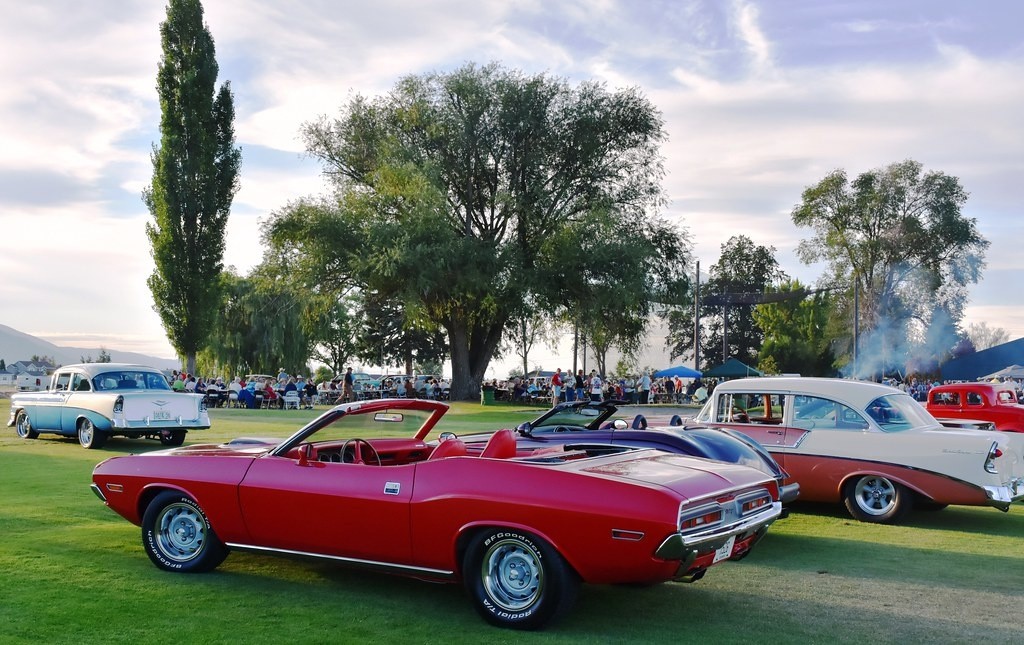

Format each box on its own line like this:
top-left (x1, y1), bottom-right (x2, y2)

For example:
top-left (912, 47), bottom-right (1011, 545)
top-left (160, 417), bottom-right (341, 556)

top-left (654, 366), bottom-right (702, 377)
top-left (977, 365), bottom-right (1024, 380)
top-left (703, 357), bottom-right (764, 377)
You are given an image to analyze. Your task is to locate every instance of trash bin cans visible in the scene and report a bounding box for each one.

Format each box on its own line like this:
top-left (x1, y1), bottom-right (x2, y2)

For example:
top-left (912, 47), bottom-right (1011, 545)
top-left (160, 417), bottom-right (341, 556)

top-left (480, 386), bottom-right (495, 405)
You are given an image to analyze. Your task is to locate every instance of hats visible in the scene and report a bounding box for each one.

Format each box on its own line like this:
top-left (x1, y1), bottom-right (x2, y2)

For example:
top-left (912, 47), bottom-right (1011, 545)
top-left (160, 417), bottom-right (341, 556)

top-left (278, 367), bottom-right (286, 372)
top-left (296, 375), bottom-right (304, 379)
top-left (674, 375), bottom-right (678, 379)
top-left (347, 367), bottom-right (354, 370)
top-left (332, 380), bottom-right (338, 382)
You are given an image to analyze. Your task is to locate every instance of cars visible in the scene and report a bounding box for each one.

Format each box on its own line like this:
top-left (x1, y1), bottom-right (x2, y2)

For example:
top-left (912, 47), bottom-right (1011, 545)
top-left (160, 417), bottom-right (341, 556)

top-left (577, 372), bottom-right (1024, 525)
top-left (377, 374), bottom-right (451, 395)
top-left (919, 382), bottom-right (1024, 434)
top-left (6, 362), bottom-right (211, 450)
top-left (316, 373), bottom-right (381, 393)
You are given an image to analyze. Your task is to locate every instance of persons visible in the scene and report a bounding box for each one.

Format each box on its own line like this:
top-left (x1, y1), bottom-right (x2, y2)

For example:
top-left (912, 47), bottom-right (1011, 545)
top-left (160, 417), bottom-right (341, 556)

top-left (485, 368), bottom-right (766, 408)
top-left (135, 373), bottom-right (165, 389)
top-left (881, 377), bottom-right (1024, 405)
top-left (167, 367), bottom-right (452, 409)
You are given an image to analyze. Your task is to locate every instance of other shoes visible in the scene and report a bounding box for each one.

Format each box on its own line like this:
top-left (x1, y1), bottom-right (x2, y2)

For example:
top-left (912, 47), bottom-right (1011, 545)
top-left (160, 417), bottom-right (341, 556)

top-left (304, 406), bottom-right (313, 410)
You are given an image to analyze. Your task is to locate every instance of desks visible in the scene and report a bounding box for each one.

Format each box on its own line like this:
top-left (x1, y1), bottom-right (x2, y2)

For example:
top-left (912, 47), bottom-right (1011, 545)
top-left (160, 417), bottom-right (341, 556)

top-left (560, 389), bottom-right (586, 402)
top-left (497, 388), bottom-right (510, 392)
top-left (656, 393), bottom-right (676, 403)
top-left (318, 389), bottom-right (388, 404)
top-left (255, 389), bottom-right (306, 410)
top-left (203, 390), bottom-right (229, 407)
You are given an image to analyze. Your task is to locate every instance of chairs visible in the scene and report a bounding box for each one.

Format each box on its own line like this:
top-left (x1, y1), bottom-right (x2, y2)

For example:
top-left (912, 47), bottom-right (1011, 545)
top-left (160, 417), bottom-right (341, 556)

top-left (427, 438), bottom-right (467, 461)
top-left (173, 388), bottom-right (450, 411)
top-left (114, 380), bottom-right (141, 389)
top-left (76, 380), bottom-right (90, 391)
top-left (670, 415), bottom-right (683, 426)
top-left (632, 414), bottom-right (647, 429)
top-left (480, 429), bottom-right (517, 458)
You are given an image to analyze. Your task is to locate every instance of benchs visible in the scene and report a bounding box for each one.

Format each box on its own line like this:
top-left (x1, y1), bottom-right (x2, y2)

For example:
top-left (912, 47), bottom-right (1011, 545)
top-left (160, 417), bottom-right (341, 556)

top-left (530, 397), bottom-right (554, 404)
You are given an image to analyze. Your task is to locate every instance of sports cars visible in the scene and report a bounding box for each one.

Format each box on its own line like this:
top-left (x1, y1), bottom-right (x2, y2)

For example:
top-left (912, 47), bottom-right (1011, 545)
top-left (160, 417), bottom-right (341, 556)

top-left (426, 398), bottom-right (807, 506)
top-left (91, 396), bottom-right (783, 632)
